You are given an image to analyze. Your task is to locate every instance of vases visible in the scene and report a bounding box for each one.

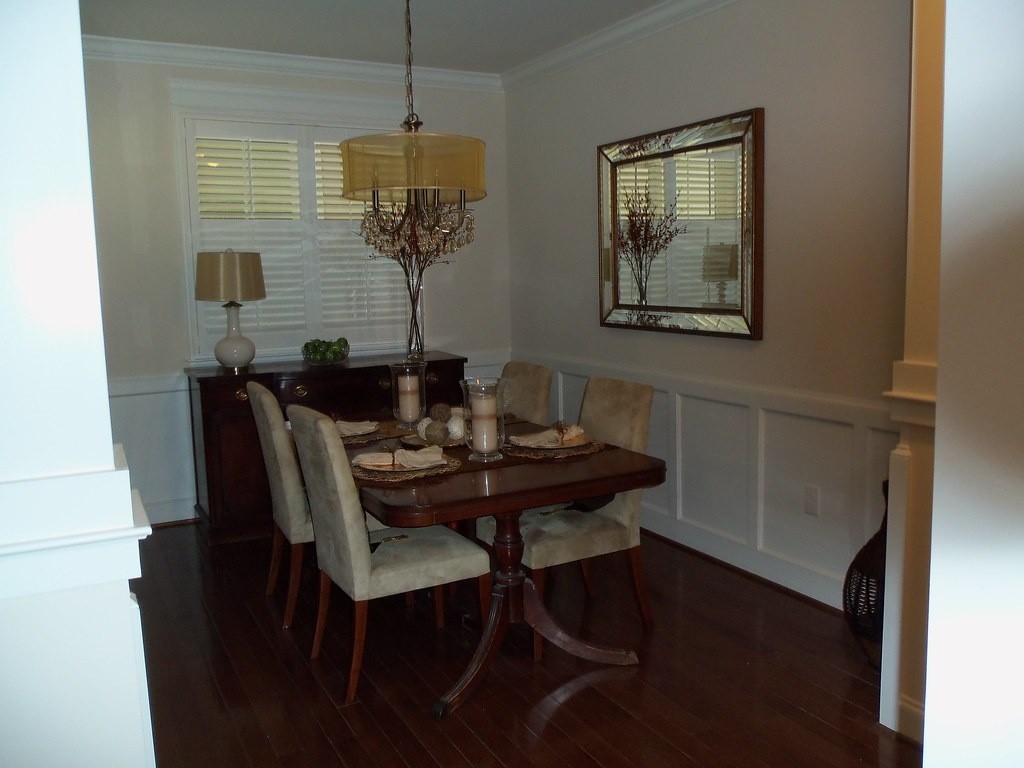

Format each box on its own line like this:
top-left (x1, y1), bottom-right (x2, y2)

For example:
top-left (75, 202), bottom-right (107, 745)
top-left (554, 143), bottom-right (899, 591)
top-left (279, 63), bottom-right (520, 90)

top-left (404, 275), bottom-right (427, 361)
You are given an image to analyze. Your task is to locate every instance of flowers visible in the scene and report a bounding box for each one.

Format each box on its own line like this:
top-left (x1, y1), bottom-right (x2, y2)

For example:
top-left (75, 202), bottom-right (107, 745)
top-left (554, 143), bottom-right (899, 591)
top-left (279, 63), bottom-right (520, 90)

top-left (608, 177), bottom-right (689, 305)
top-left (353, 201), bottom-right (476, 356)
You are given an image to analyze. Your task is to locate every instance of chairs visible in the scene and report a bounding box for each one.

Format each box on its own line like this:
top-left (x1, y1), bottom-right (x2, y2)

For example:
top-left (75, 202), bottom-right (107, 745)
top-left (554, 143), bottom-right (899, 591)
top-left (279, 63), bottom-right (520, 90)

top-left (247, 380), bottom-right (412, 634)
top-left (435, 357), bottom-right (556, 630)
top-left (285, 403), bottom-right (491, 710)
top-left (469, 374), bottom-right (658, 665)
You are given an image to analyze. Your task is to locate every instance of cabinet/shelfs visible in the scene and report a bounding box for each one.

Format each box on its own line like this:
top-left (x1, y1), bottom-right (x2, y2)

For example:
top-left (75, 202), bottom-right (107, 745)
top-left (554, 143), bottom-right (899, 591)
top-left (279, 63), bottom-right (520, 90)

top-left (183, 349), bottom-right (469, 548)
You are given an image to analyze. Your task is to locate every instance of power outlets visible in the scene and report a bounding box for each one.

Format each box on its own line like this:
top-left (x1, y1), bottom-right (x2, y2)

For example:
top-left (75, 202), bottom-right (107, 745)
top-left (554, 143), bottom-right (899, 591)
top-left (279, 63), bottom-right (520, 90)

top-left (803, 484), bottom-right (821, 519)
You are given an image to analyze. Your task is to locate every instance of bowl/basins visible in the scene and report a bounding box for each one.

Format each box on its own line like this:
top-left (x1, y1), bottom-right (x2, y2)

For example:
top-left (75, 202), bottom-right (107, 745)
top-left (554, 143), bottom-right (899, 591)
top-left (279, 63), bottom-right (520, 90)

top-left (302, 345), bottom-right (350, 365)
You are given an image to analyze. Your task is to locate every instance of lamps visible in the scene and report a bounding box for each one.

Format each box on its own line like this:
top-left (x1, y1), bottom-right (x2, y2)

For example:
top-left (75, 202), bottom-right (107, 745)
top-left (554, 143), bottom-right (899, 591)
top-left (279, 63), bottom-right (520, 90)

top-left (335, 0), bottom-right (489, 238)
top-left (194, 248), bottom-right (268, 371)
top-left (701, 244), bottom-right (740, 310)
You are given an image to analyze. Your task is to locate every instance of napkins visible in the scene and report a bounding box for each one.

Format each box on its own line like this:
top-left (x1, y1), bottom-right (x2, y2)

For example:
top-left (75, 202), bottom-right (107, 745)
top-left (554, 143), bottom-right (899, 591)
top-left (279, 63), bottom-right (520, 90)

top-left (333, 421), bottom-right (379, 438)
top-left (353, 444), bottom-right (449, 467)
top-left (506, 424), bottom-right (584, 448)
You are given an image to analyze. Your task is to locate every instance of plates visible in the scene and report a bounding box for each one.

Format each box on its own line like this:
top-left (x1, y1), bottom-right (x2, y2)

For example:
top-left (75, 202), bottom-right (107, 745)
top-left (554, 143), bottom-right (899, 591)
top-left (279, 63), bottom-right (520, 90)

top-left (451, 407), bottom-right (471, 420)
top-left (400, 434), bottom-right (469, 448)
top-left (340, 426), bottom-right (379, 437)
top-left (511, 435), bottom-right (590, 449)
top-left (359, 463), bottom-right (425, 471)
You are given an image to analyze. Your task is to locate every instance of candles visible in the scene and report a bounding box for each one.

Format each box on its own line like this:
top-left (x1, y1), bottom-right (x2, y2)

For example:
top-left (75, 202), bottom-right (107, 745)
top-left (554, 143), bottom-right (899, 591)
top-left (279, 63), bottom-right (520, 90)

top-left (469, 379), bottom-right (498, 454)
top-left (398, 375), bottom-right (420, 423)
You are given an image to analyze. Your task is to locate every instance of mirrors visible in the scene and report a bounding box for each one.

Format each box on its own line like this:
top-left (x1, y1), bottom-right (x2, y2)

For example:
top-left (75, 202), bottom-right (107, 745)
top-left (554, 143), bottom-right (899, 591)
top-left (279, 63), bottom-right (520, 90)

top-left (596, 106), bottom-right (765, 343)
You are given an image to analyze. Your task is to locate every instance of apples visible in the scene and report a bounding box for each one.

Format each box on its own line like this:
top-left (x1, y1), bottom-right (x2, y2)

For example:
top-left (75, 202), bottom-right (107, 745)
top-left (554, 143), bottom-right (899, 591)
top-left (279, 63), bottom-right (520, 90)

top-left (305, 338), bottom-right (348, 362)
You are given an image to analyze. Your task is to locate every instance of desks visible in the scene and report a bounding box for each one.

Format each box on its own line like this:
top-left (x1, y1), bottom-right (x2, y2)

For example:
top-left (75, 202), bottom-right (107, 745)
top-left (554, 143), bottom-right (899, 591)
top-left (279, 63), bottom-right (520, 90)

top-left (345, 420), bottom-right (669, 719)
